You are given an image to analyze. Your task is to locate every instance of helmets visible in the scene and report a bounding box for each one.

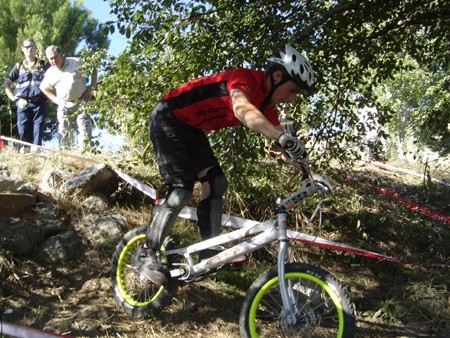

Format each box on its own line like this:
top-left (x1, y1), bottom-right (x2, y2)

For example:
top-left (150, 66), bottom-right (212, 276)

top-left (266, 44), bottom-right (313, 92)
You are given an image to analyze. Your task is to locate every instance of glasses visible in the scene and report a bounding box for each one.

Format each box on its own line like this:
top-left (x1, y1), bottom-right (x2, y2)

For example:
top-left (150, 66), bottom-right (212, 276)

top-left (22, 44), bottom-right (35, 48)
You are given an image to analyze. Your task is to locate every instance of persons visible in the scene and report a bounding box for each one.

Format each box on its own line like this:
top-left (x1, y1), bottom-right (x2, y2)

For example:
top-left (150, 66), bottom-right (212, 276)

top-left (39, 45), bottom-right (97, 151)
top-left (132, 44), bottom-right (314, 286)
top-left (4, 39), bottom-right (51, 149)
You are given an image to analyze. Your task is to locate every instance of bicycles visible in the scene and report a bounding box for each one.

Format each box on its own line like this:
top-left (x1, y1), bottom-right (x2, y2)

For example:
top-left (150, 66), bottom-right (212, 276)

top-left (111, 140), bottom-right (355, 338)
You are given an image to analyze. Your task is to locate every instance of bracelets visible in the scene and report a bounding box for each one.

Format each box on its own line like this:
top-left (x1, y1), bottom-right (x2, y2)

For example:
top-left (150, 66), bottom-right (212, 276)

top-left (90, 86), bottom-right (95, 89)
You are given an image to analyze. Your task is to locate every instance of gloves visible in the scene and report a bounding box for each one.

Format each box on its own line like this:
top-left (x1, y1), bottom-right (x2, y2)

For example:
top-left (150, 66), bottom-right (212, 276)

top-left (277, 132), bottom-right (304, 157)
top-left (301, 174), bottom-right (333, 195)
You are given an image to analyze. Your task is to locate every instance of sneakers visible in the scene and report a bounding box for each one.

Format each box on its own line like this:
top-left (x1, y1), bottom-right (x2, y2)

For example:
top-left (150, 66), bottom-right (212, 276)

top-left (198, 248), bottom-right (248, 264)
top-left (131, 244), bottom-right (170, 285)
